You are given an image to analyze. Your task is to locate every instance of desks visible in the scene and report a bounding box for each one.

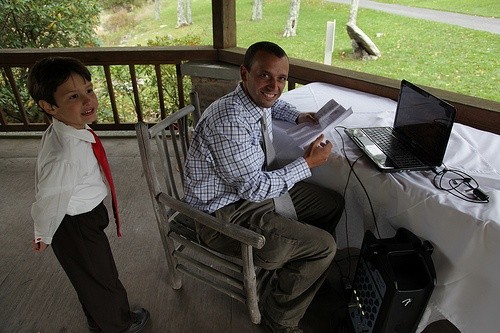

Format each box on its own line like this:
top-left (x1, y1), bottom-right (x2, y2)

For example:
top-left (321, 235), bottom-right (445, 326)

top-left (272, 82), bottom-right (500, 333)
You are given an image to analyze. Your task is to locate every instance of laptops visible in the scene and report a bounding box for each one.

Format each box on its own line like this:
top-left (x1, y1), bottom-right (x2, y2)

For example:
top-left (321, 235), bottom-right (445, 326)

top-left (344, 79), bottom-right (457, 173)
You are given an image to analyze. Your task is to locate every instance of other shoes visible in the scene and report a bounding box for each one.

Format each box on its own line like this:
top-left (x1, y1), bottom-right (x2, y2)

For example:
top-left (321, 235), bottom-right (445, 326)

top-left (258, 303), bottom-right (304, 333)
top-left (121, 308), bottom-right (150, 333)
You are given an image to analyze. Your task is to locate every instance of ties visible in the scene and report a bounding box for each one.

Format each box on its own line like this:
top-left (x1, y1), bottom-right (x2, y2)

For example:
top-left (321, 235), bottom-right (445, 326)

top-left (261, 112), bottom-right (298, 220)
top-left (88, 129), bottom-right (121, 237)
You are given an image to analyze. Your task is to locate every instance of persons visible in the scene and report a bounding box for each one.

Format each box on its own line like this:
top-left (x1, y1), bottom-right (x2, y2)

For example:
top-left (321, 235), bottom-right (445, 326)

top-left (26, 56), bottom-right (153, 333)
top-left (182, 41), bottom-right (346, 333)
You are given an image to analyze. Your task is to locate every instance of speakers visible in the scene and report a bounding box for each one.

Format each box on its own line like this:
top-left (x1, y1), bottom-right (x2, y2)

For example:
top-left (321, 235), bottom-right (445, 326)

top-left (343, 228), bottom-right (437, 333)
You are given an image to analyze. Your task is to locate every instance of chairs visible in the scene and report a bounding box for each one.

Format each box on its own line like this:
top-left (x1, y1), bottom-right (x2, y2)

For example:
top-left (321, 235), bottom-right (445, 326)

top-left (135, 92), bottom-right (336, 324)
